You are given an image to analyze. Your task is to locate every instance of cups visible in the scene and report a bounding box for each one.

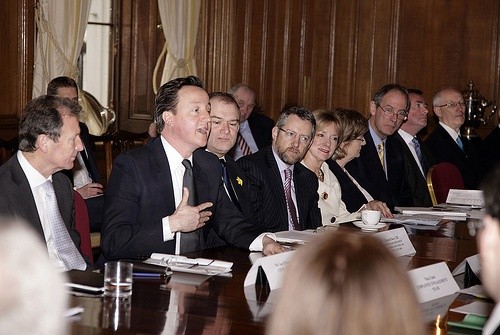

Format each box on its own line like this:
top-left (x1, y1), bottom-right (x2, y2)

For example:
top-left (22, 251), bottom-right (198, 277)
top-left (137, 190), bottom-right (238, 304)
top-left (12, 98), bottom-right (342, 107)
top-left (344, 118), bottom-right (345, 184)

top-left (101, 296), bottom-right (132, 330)
top-left (362, 210), bottom-right (380, 226)
top-left (102, 260), bottom-right (133, 296)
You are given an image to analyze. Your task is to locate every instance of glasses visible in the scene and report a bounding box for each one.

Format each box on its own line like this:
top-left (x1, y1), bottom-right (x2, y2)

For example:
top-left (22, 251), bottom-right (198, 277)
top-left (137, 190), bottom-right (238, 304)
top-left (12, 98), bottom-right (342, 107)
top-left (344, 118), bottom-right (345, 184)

top-left (437, 100), bottom-right (466, 108)
top-left (353, 138), bottom-right (365, 143)
top-left (377, 104), bottom-right (408, 121)
top-left (278, 127), bottom-right (311, 144)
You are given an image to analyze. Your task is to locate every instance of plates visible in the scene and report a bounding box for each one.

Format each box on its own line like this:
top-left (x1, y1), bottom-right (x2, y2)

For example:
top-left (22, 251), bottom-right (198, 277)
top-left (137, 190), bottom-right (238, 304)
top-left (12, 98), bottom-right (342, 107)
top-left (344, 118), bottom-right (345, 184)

top-left (352, 221), bottom-right (387, 232)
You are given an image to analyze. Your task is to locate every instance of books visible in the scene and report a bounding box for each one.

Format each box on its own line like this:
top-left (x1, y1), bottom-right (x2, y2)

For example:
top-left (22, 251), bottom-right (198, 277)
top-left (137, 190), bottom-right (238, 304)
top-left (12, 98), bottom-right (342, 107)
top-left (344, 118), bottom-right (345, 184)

top-left (64, 269), bottom-right (104, 297)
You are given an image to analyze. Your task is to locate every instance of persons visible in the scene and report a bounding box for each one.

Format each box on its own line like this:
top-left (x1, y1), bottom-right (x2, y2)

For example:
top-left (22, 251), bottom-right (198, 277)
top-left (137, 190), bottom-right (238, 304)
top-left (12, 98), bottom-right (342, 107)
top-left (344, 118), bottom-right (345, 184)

top-left (0, 95), bottom-right (92, 272)
top-left (391, 88), bottom-right (438, 208)
top-left (236, 105), bottom-right (323, 233)
top-left (325, 107), bottom-right (376, 214)
top-left (47, 77), bottom-right (104, 233)
top-left (420, 87), bottom-right (479, 188)
top-left (195, 92), bottom-right (256, 249)
top-left (265, 228), bottom-right (426, 335)
top-left (475, 166), bottom-right (500, 335)
top-left (300, 110), bottom-right (394, 227)
top-left (476, 106), bottom-right (500, 178)
top-left (95, 76), bottom-right (289, 268)
top-left (225, 82), bottom-right (277, 162)
top-left (360, 84), bottom-right (414, 214)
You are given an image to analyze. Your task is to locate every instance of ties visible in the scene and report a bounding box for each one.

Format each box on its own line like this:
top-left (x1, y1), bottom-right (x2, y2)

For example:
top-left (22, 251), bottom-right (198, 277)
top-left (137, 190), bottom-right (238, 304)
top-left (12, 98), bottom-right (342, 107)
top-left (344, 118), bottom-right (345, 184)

top-left (412, 137), bottom-right (428, 178)
top-left (378, 141), bottom-right (385, 171)
top-left (284, 168), bottom-right (300, 231)
top-left (456, 135), bottom-right (464, 150)
top-left (79, 150), bottom-right (94, 176)
top-left (38, 180), bottom-right (85, 272)
top-left (180, 158), bottom-right (200, 254)
top-left (220, 158), bottom-right (245, 216)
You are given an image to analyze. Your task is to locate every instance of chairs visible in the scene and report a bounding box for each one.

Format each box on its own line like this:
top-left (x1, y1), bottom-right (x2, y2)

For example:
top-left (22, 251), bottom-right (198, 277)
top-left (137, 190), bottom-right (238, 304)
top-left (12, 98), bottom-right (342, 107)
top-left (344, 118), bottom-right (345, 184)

top-left (428, 163), bottom-right (463, 207)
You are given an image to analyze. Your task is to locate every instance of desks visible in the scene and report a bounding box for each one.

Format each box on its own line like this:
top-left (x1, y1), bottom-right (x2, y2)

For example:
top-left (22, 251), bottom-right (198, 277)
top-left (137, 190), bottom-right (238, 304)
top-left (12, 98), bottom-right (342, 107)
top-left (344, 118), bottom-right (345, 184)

top-left (70, 219), bottom-right (477, 335)
